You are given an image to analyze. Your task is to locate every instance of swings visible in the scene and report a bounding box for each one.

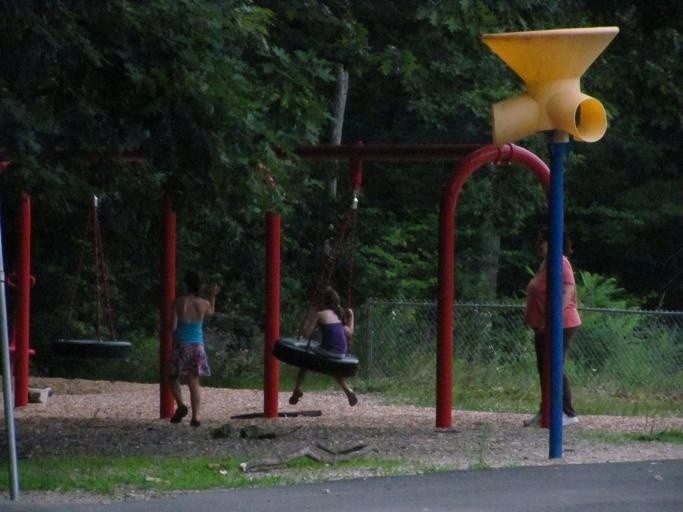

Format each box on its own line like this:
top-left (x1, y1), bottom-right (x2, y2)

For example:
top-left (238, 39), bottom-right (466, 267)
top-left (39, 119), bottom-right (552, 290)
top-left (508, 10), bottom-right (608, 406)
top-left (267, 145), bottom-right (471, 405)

top-left (271, 193), bottom-right (361, 379)
top-left (48, 195), bottom-right (132, 359)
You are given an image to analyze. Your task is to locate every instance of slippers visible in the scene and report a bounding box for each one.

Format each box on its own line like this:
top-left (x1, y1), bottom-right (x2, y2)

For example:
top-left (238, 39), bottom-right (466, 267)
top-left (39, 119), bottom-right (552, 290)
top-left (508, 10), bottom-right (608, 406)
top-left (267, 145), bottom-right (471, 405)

top-left (169, 406), bottom-right (188, 423)
top-left (191, 418), bottom-right (200, 427)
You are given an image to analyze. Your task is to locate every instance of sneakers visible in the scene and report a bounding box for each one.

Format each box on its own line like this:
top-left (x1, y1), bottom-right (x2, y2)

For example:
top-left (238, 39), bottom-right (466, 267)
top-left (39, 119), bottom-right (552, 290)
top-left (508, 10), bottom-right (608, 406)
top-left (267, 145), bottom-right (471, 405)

top-left (289, 387), bottom-right (304, 405)
top-left (562, 412), bottom-right (579, 426)
top-left (522, 414), bottom-right (543, 427)
top-left (345, 389), bottom-right (360, 407)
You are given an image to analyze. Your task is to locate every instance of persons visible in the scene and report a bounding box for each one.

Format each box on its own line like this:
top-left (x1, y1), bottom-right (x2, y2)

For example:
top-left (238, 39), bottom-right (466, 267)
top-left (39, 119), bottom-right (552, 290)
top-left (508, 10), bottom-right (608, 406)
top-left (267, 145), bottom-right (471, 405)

top-left (289, 287), bottom-right (358, 406)
top-left (168, 269), bottom-right (221, 426)
top-left (523, 226), bottom-right (583, 427)
